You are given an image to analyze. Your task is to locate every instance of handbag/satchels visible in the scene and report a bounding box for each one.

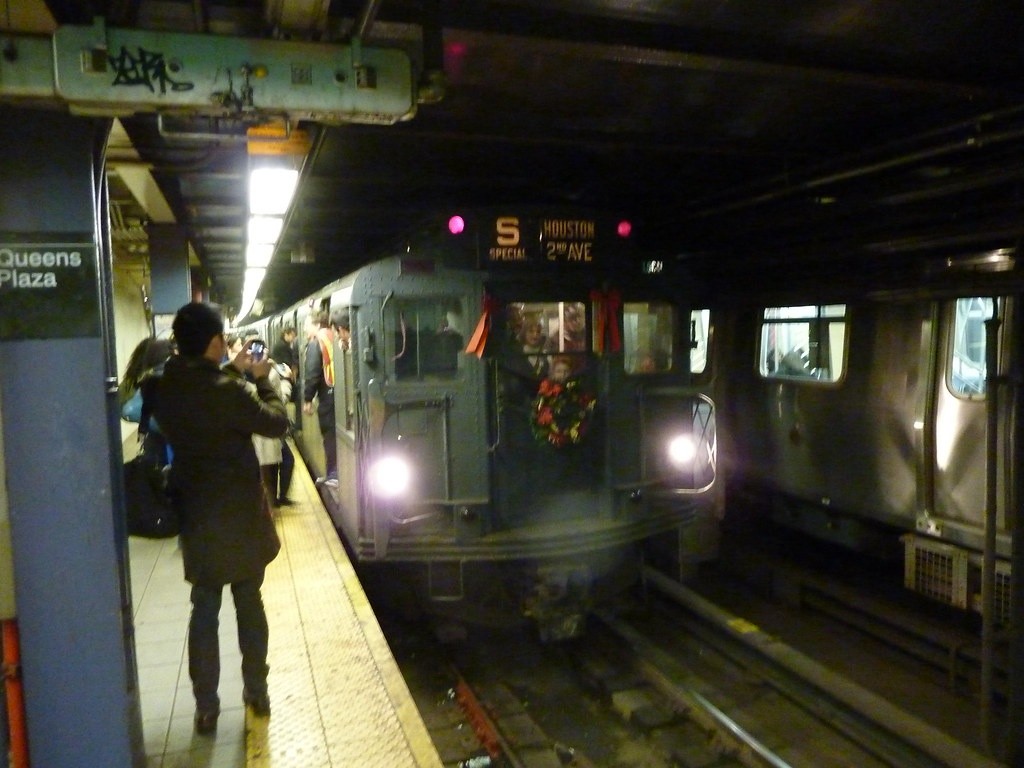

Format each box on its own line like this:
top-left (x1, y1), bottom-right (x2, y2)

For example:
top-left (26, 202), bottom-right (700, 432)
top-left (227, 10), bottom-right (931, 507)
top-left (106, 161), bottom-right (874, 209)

top-left (120, 456), bottom-right (182, 539)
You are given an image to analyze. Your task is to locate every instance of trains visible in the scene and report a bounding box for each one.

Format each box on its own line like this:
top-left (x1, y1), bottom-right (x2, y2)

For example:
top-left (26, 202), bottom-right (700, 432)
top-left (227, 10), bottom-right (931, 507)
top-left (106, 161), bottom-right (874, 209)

top-left (713, 124), bottom-right (1019, 625)
top-left (224, 206), bottom-right (725, 647)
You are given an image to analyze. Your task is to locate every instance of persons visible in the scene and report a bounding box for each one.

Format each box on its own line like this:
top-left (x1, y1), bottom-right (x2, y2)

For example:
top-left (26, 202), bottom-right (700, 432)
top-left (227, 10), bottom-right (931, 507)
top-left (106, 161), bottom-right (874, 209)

top-left (117, 321), bottom-right (259, 472)
top-left (495, 307), bottom-right (549, 514)
top-left (766, 348), bottom-right (810, 376)
top-left (271, 327), bottom-right (299, 436)
top-left (138, 301), bottom-right (286, 736)
top-left (553, 356), bottom-right (570, 384)
top-left (549, 303), bottom-right (588, 366)
top-left (329, 309), bottom-right (349, 349)
top-left (242, 341), bottom-right (295, 508)
top-left (642, 354), bottom-right (668, 375)
top-left (515, 322), bottom-right (552, 373)
top-left (303, 311), bottom-right (339, 488)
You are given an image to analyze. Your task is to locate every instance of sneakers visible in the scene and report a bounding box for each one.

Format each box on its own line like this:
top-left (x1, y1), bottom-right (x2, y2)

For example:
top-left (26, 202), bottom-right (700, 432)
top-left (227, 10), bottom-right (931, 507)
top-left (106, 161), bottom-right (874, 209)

top-left (191, 695), bottom-right (222, 731)
top-left (240, 664), bottom-right (271, 717)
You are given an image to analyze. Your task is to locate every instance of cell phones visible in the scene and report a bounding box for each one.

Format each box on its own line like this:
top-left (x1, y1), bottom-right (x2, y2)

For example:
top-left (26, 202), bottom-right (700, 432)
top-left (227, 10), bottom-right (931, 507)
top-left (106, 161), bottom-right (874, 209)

top-left (249, 339), bottom-right (265, 363)
